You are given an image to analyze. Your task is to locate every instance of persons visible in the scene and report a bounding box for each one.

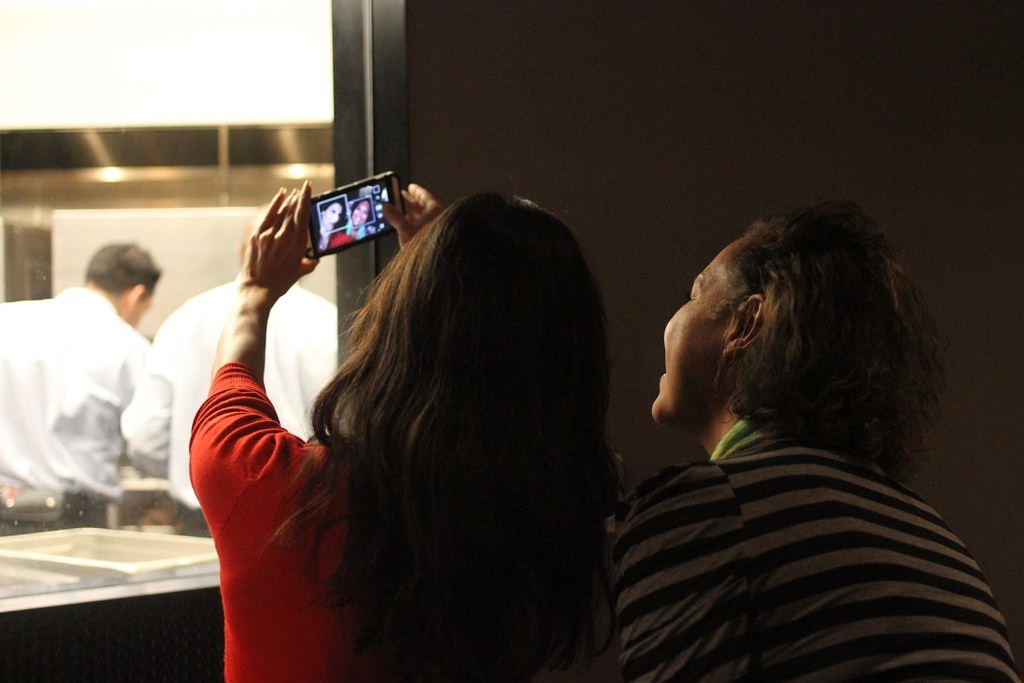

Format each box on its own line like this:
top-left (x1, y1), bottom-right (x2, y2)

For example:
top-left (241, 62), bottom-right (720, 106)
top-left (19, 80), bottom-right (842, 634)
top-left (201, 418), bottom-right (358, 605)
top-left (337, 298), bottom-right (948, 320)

top-left (122, 202), bottom-right (338, 539)
top-left (191, 181), bottom-right (620, 683)
top-left (0, 244), bottom-right (159, 536)
top-left (318, 200), bottom-right (355, 251)
top-left (345, 199), bottom-right (371, 240)
top-left (607, 199), bottom-right (1024, 683)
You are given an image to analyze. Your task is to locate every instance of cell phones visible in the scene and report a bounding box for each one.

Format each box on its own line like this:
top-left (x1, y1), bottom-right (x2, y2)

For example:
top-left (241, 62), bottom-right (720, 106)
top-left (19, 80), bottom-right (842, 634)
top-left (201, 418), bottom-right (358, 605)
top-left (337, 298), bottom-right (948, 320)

top-left (303, 170), bottom-right (408, 259)
